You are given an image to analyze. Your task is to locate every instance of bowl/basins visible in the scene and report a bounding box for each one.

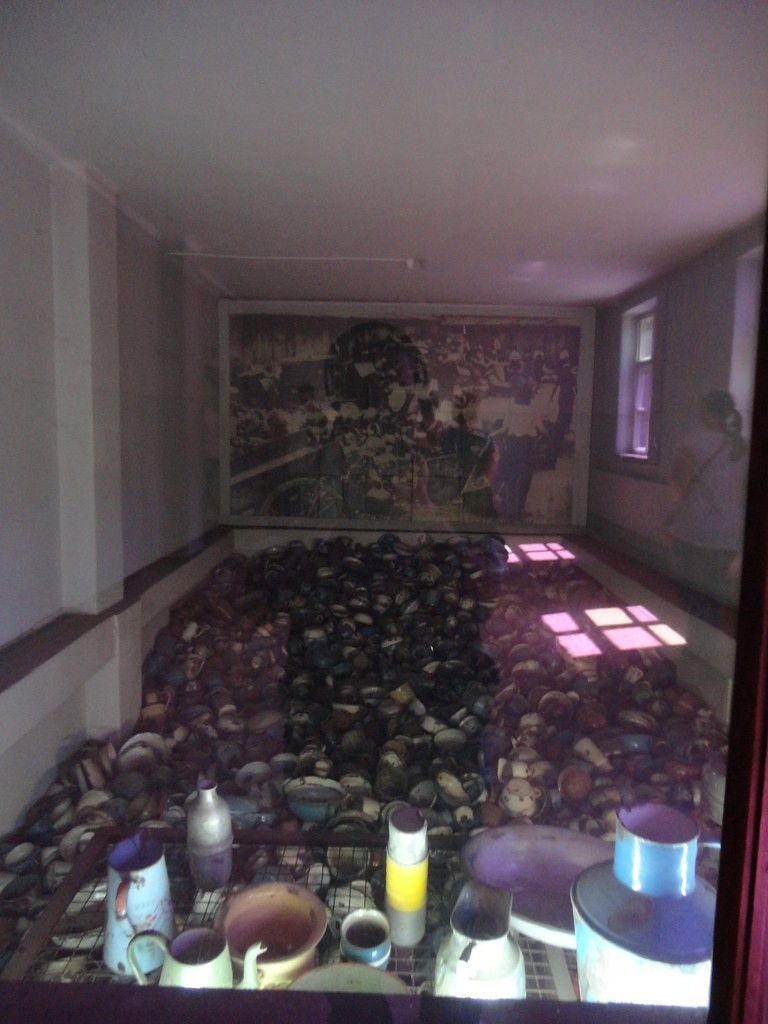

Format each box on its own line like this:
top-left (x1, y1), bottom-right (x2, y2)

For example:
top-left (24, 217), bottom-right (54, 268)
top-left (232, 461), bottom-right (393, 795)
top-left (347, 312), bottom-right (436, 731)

top-left (0, 533), bottom-right (727, 983)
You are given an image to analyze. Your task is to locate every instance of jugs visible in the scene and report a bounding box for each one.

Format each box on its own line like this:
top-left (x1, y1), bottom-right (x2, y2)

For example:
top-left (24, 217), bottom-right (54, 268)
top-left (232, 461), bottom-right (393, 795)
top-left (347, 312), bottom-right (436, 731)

top-left (102, 827), bottom-right (174, 973)
top-left (432, 884), bottom-right (525, 999)
top-left (128, 928), bottom-right (266, 992)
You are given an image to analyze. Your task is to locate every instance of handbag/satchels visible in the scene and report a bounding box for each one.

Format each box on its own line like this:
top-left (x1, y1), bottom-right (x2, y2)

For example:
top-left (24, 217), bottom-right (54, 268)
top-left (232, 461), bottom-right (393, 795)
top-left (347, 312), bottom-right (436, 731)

top-left (663, 501), bottom-right (686, 536)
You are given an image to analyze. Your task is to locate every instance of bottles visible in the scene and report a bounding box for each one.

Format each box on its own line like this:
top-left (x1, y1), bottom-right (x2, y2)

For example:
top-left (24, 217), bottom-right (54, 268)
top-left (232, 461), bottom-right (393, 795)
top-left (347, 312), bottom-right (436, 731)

top-left (569, 805), bottom-right (722, 1007)
top-left (190, 779), bottom-right (234, 889)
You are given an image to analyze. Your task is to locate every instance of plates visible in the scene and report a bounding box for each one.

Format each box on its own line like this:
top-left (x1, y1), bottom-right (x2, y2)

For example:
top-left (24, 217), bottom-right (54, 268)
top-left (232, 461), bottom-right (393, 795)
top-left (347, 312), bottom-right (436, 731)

top-left (463, 826), bottom-right (616, 950)
top-left (293, 964), bottom-right (411, 994)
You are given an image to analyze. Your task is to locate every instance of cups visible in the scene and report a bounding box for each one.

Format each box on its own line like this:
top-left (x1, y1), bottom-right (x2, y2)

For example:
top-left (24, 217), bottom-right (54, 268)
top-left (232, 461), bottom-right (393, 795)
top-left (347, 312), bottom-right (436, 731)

top-left (340, 909), bottom-right (392, 971)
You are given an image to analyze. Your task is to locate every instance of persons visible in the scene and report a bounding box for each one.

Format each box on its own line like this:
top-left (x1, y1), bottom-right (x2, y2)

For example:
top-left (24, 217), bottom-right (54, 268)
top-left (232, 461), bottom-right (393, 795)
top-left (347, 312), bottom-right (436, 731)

top-left (232, 313), bottom-right (581, 523)
top-left (649, 386), bottom-right (749, 600)
